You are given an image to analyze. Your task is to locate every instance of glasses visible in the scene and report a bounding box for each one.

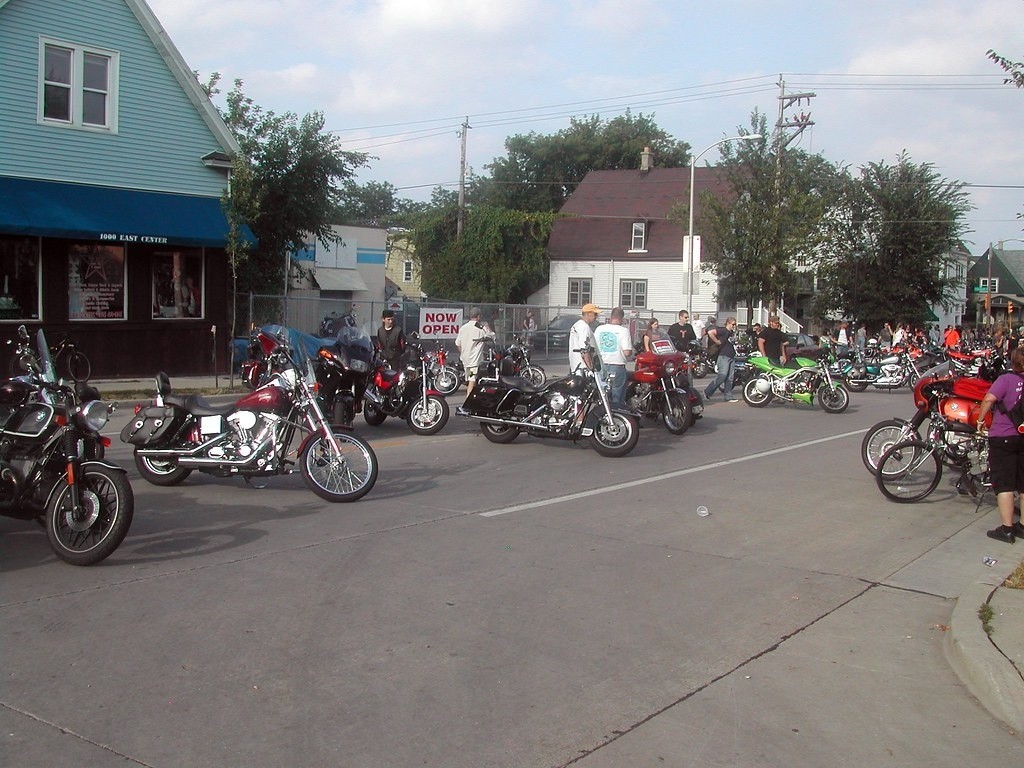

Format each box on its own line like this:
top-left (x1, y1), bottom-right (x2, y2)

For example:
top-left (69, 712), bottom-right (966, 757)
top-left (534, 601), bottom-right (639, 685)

top-left (732, 324), bottom-right (736, 326)
top-left (685, 316), bottom-right (689, 319)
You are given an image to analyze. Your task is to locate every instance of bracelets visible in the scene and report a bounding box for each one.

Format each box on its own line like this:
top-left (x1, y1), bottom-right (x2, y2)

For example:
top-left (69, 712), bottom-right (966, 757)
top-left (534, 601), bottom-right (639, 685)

top-left (977, 419), bottom-right (985, 425)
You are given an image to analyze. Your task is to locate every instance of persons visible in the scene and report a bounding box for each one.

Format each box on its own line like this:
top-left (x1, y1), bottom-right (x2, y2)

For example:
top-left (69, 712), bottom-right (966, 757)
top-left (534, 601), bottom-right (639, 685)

top-left (857, 323), bottom-right (868, 349)
top-left (746, 323), bottom-right (767, 347)
top-left (667, 310), bottom-right (696, 352)
top-left (455, 306), bottom-right (497, 397)
top-left (377, 310), bottom-right (406, 371)
top-left (642, 317), bottom-right (661, 352)
top-left (977, 348), bottom-right (1024, 543)
top-left (756, 316), bottom-right (790, 365)
top-left (523, 311), bottom-right (537, 346)
top-left (568, 304), bottom-right (633, 421)
top-left (819, 320), bottom-right (853, 356)
top-left (877, 322), bottom-right (1024, 369)
top-left (692, 313), bottom-right (719, 348)
top-left (703, 316), bottom-right (739, 403)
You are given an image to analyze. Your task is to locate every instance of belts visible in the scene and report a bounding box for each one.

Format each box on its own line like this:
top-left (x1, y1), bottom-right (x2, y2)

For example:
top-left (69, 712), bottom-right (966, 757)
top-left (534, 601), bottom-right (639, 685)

top-left (604, 364), bottom-right (624, 366)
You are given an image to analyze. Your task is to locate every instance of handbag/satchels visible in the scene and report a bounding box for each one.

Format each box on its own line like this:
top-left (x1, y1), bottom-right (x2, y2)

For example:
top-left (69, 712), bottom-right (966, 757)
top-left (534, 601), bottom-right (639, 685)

top-left (708, 344), bottom-right (719, 361)
top-left (996, 373), bottom-right (1024, 435)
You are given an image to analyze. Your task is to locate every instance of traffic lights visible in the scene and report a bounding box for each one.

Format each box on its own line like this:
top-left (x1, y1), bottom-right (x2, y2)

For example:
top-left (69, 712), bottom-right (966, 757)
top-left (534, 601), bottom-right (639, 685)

top-left (1008, 301), bottom-right (1013, 314)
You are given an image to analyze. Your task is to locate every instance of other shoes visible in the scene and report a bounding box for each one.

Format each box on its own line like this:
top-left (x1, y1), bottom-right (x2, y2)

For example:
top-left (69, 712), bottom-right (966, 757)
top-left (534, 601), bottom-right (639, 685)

top-left (725, 399), bottom-right (738, 402)
top-left (704, 390), bottom-right (710, 400)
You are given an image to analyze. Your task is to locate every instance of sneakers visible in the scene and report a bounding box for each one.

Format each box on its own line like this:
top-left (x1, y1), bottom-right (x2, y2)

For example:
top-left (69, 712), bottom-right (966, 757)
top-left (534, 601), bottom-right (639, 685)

top-left (1013, 521), bottom-right (1024, 539)
top-left (987, 526), bottom-right (1015, 543)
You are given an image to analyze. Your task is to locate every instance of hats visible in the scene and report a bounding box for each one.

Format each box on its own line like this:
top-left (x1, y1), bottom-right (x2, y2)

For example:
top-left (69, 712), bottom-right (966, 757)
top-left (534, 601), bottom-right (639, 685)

top-left (1016, 326), bottom-right (1024, 331)
top-left (770, 316), bottom-right (780, 323)
top-left (582, 303), bottom-right (603, 313)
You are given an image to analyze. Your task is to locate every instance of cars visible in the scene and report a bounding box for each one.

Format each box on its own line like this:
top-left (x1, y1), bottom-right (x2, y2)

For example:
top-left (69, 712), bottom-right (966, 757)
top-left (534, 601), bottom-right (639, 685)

top-left (532, 316), bottom-right (603, 351)
top-left (386, 299), bottom-right (420, 334)
top-left (780, 332), bottom-right (821, 361)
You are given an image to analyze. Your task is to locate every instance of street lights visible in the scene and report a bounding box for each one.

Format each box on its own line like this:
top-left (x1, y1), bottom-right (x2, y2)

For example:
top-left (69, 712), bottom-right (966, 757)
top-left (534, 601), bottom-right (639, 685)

top-left (986, 239), bottom-right (1024, 329)
top-left (687, 134), bottom-right (763, 328)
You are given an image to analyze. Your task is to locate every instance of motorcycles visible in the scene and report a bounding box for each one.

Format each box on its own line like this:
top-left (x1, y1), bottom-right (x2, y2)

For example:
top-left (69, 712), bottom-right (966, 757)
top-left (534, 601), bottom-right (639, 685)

top-left (454, 330), bottom-right (642, 459)
top-left (821, 334), bottom-right (1024, 513)
top-left (0, 324), bottom-right (135, 567)
top-left (446, 333), bottom-right (547, 391)
top-left (241, 304), bottom-right (461, 443)
top-left (119, 319), bottom-right (379, 503)
top-left (624, 327), bottom-right (849, 435)
